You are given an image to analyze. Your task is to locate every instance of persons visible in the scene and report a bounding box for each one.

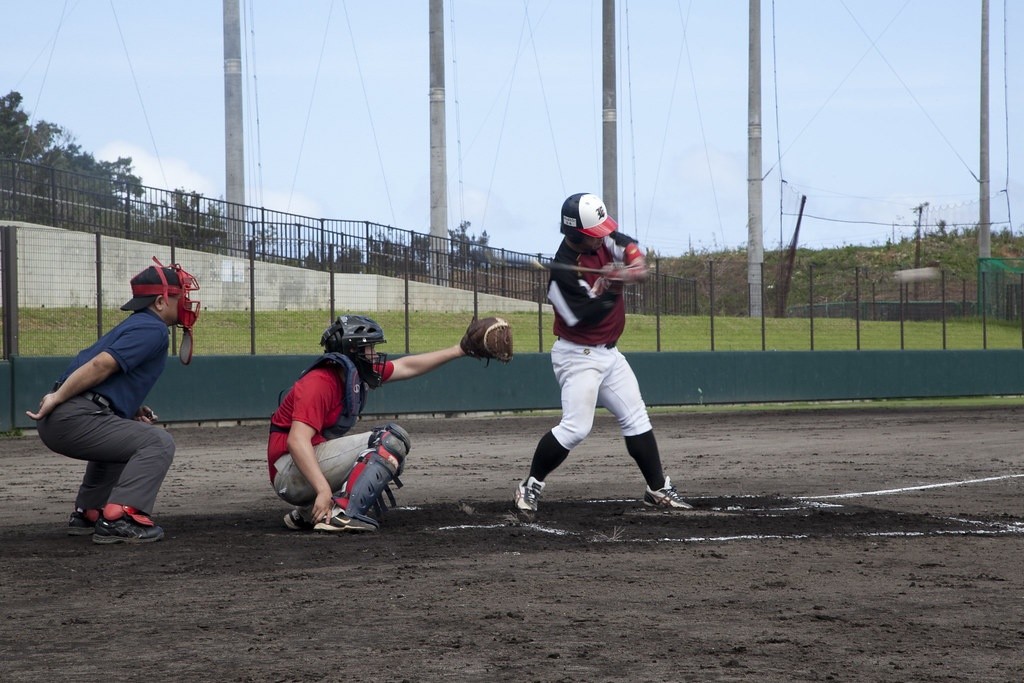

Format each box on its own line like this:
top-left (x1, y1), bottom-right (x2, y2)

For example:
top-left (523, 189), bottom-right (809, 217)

top-left (512, 194), bottom-right (692, 510)
top-left (266, 314), bottom-right (512, 533)
top-left (24, 256), bottom-right (202, 544)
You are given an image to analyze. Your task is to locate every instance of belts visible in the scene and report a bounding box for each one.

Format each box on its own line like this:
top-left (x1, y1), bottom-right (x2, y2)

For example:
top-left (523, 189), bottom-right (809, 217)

top-left (589, 341), bottom-right (617, 349)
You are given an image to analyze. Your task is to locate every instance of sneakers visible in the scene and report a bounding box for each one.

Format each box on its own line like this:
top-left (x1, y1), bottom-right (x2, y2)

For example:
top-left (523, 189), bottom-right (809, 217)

top-left (513, 477), bottom-right (546, 512)
top-left (643, 475), bottom-right (694, 508)
top-left (314, 507), bottom-right (378, 532)
top-left (68, 511), bottom-right (96, 535)
top-left (284, 506), bottom-right (314, 531)
top-left (92, 519), bottom-right (165, 545)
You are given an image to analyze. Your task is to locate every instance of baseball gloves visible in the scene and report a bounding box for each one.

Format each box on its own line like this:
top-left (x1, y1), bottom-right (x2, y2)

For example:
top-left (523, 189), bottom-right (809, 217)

top-left (460, 314), bottom-right (514, 368)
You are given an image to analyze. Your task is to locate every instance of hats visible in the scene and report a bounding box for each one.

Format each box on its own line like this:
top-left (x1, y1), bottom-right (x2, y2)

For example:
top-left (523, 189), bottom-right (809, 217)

top-left (120, 266), bottom-right (183, 311)
top-left (561, 193), bottom-right (619, 238)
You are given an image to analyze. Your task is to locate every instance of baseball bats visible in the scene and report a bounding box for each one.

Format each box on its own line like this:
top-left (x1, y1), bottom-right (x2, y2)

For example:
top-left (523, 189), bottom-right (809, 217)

top-left (541, 262), bottom-right (607, 274)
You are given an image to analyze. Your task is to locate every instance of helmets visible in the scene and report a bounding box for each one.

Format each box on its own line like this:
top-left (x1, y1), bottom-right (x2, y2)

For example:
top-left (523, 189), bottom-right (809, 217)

top-left (320, 315), bottom-right (387, 391)
